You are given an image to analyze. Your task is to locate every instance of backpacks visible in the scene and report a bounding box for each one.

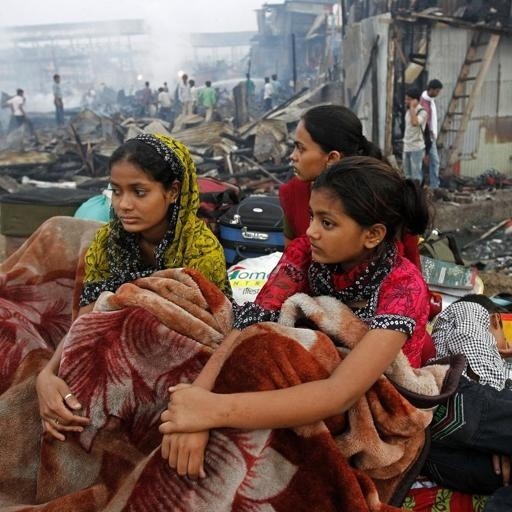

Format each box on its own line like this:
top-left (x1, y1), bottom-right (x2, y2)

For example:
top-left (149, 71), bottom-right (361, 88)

top-left (416, 109), bottom-right (431, 154)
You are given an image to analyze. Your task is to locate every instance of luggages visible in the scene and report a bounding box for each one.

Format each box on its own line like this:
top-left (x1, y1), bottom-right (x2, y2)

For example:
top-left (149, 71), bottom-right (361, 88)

top-left (219, 194), bottom-right (285, 268)
top-left (197, 176), bottom-right (240, 238)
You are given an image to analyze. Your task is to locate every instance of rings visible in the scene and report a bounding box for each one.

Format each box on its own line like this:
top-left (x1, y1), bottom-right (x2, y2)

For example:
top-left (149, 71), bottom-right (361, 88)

top-left (53, 415), bottom-right (64, 425)
top-left (64, 393), bottom-right (74, 400)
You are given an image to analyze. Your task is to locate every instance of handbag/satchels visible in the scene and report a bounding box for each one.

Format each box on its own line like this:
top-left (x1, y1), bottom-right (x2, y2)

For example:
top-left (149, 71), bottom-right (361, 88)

top-left (418, 231), bottom-right (465, 266)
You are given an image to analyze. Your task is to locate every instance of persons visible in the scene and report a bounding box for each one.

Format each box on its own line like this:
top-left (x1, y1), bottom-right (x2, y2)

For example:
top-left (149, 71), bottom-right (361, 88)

top-left (275, 103), bottom-right (423, 271)
top-left (418, 77), bottom-right (444, 191)
top-left (400, 87), bottom-right (429, 191)
top-left (34, 131), bottom-right (234, 443)
top-left (417, 293), bottom-right (512, 511)
top-left (156, 154), bottom-right (432, 480)
top-left (76, 69), bottom-right (282, 130)
top-left (0, 88), bottom-right (27, 137)
top-left (50, 72), bottom-right (70, 128)
